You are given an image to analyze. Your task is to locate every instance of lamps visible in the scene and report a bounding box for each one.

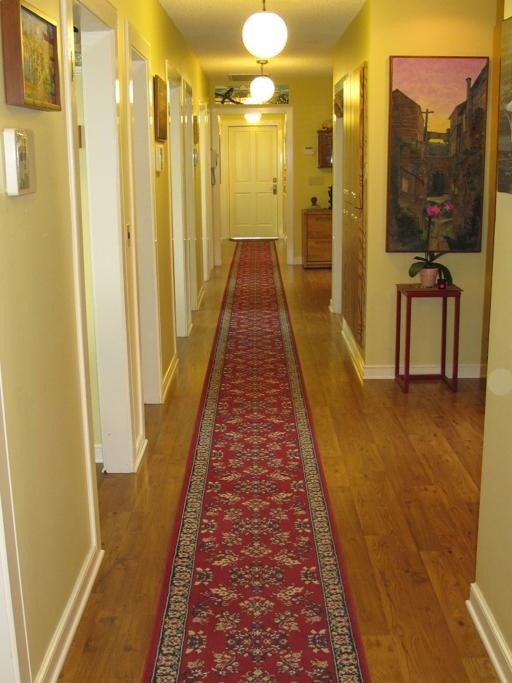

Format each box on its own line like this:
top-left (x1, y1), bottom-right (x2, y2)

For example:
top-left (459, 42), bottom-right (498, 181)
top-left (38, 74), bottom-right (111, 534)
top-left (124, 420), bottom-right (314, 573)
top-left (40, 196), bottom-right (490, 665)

top-left (241, 0), bottom-right (289, 62)
top-left (248, 59), bottom-right (275, 103)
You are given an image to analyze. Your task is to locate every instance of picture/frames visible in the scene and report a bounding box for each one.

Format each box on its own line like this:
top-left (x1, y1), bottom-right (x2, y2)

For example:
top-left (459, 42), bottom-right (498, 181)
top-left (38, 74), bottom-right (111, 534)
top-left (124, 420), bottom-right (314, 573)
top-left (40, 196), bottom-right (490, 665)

top-left (385, 54), bottom-right (490, 254)
top-left (192, 115), bottom-right (200, 145)
top-left (1, 1), bottom-right (62, 114)
top-left (153, 74), bottom-right (169, 144)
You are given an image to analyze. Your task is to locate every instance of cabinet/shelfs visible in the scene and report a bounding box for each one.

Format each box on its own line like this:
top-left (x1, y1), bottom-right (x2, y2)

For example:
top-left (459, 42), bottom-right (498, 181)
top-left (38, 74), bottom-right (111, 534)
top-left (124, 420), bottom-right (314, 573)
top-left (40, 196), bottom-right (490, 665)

top-left (300, 207), bottom-right (336, 270)
top-left (341, 60), bottom-right (368, 356)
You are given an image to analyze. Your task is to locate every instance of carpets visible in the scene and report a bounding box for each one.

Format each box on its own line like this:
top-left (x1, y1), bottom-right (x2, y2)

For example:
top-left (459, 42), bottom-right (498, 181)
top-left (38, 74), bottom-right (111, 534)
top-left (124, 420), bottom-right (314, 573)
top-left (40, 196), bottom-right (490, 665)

top-left (141, 239), bottom-right (371, 682)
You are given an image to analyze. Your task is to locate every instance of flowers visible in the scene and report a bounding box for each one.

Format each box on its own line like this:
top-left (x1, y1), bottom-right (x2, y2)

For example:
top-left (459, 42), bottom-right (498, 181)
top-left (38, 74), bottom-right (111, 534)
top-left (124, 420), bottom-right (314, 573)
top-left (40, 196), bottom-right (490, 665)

top-left (409, 200), bottom-right (456, 290)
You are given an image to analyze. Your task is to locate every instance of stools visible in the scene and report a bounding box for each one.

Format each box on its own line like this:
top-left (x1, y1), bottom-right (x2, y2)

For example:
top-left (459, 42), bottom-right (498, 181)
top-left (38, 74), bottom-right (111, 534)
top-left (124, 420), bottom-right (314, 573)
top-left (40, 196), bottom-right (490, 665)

top-left (394, 281), bottom-right (463, 394)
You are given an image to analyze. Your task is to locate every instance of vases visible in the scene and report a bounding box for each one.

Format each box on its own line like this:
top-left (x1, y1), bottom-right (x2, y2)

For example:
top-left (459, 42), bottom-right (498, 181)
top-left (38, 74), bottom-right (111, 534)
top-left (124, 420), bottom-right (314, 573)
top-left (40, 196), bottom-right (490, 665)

top-left (419, 268), bottom-right (439, 288)
